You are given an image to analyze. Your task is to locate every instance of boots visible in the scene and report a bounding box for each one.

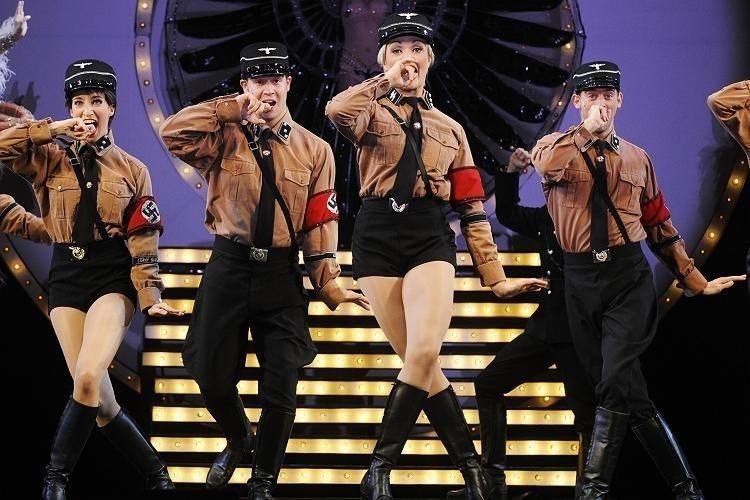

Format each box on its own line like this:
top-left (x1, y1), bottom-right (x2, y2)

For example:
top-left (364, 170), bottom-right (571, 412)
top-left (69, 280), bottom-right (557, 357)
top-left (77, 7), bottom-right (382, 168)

top-left (203, 388), bottom-right (257, 489)
top-left (95, 405), bottom-right (178, 496)
top-left (246, 406), bottom-right (296, 500)
top-left (360, 378), bottom-right (429, 499)
top-left (42, 396), bottom-right (102, 500)
top-left (423, 384), bottom-right (506, 500)
top-left (575, 406), bottom-right (703, 500)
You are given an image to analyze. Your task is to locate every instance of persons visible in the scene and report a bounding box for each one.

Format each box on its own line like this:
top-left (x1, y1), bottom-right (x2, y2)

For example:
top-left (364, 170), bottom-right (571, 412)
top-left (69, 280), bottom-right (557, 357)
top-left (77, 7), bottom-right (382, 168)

top-left (705, 78), bottom-right (750, 165)
top-left (158, 41), bottom-right (372, 500)
top-left (322, 9), bottom-right (549, 500)
top-left (0, 57), bottom-right (188, 500)
top-left (529, 60), bottom-right (748, 499)
top-left (0, 193), bottom-right (52, 246)
top-left (471, 145), bottom-right (596, 500)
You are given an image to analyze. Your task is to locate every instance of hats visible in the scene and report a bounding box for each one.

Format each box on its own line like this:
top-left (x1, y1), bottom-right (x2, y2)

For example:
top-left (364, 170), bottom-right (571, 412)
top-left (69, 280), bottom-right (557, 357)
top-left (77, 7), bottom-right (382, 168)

top-left (239, 41), bottom-right (292, 79)
top-left (572, 61), bottom-right (622, 94)
top-left (376, 11), bottom-right (436, 52)
top-left (63, 60), bottom-right (117, 96)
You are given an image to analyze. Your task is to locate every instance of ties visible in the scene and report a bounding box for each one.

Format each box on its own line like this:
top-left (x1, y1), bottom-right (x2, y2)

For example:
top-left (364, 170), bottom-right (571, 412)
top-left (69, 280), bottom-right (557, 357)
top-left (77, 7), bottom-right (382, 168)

top-left (254, 129), bottom-right (276, 249)
top-left (591, 140), bottom-right (609, 255)
top-left (390, 96), bottom-right (423, 206)
top-left (71, 155), bottom-right (98, 258)
